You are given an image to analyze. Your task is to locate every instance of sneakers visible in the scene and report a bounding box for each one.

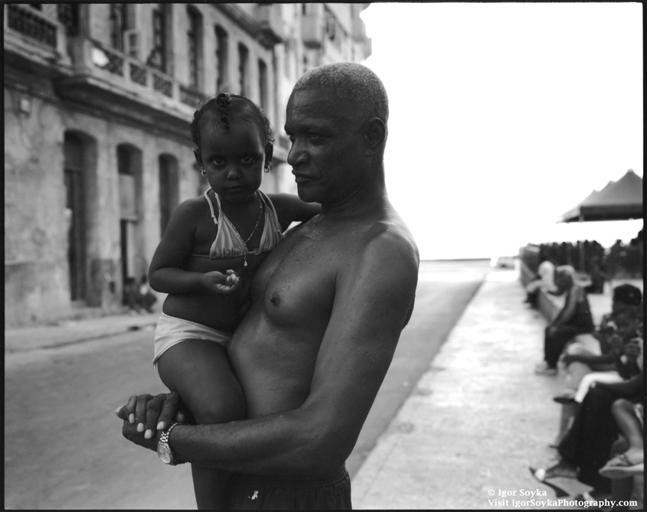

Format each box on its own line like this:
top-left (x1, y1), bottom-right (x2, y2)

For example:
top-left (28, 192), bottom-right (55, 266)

top-left (553, 391), bottom-right (575, 404)
top-left (533, 359), bottom-right (557, 376)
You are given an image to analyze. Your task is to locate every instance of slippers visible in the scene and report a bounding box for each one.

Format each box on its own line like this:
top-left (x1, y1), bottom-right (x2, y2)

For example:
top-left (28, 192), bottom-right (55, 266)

top-left (597, 453), bottom-right (645, 480)
top-left (528, 467), bottom-right (571, 497)
top-left (574, 491), bottom-right (598, 504)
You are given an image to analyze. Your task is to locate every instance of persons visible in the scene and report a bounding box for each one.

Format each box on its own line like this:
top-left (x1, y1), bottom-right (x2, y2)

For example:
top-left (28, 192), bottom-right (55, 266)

top-left (136, 273), bottom-right (157, 314)
top-left (114, 59), bottom-right (423, 512)
top-left (121, 278), bottom-right (142, 315)
top-left (147, 92), bottom-right (325, 512)
top-left (517, 227), bottom-right (646, 497)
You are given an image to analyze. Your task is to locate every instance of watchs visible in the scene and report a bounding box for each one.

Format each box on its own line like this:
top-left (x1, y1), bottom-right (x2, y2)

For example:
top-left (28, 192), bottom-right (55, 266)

top-left (155, 421), bottom-right (189, 466)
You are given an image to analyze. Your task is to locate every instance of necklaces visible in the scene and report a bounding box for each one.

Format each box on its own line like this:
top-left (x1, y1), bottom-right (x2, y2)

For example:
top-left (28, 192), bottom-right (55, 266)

top-left (208, 191), bottom-right (265, 266)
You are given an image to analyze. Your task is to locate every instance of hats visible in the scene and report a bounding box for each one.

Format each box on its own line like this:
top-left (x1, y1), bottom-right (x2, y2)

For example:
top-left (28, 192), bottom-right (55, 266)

top-left (612, 283), bottom-right (642, 306)
